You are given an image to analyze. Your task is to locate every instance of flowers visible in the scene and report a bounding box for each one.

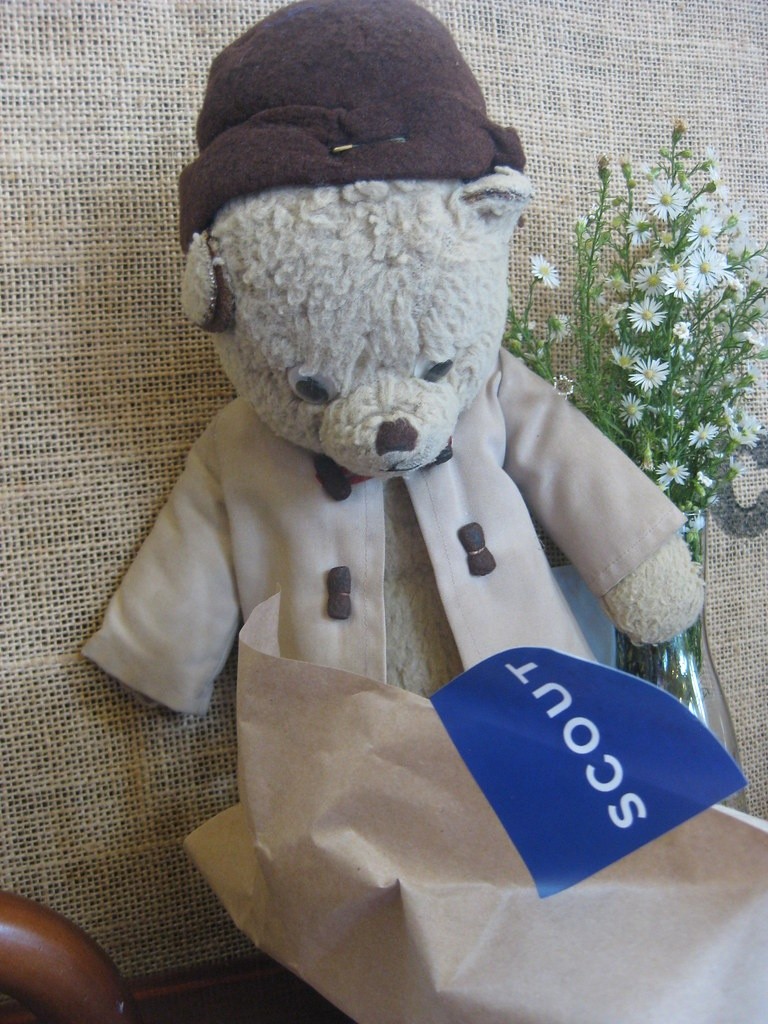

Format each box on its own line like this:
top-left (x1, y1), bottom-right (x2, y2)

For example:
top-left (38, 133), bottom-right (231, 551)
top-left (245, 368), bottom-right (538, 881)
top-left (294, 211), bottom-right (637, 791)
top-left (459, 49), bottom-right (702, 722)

top-left (503, 119), bottom-right (768, 714)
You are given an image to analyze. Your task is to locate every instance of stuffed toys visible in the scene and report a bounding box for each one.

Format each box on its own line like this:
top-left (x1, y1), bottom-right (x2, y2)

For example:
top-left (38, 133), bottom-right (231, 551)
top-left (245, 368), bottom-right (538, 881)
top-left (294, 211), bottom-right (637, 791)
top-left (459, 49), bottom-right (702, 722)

top-left (82, 1), bottom-right (704, 716)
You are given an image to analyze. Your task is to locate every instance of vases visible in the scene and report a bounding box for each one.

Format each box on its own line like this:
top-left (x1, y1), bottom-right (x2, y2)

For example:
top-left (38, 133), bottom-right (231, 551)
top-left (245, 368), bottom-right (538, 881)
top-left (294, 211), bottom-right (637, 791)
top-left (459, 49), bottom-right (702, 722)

top-left (614, 507), bottom-right (751, 816)
